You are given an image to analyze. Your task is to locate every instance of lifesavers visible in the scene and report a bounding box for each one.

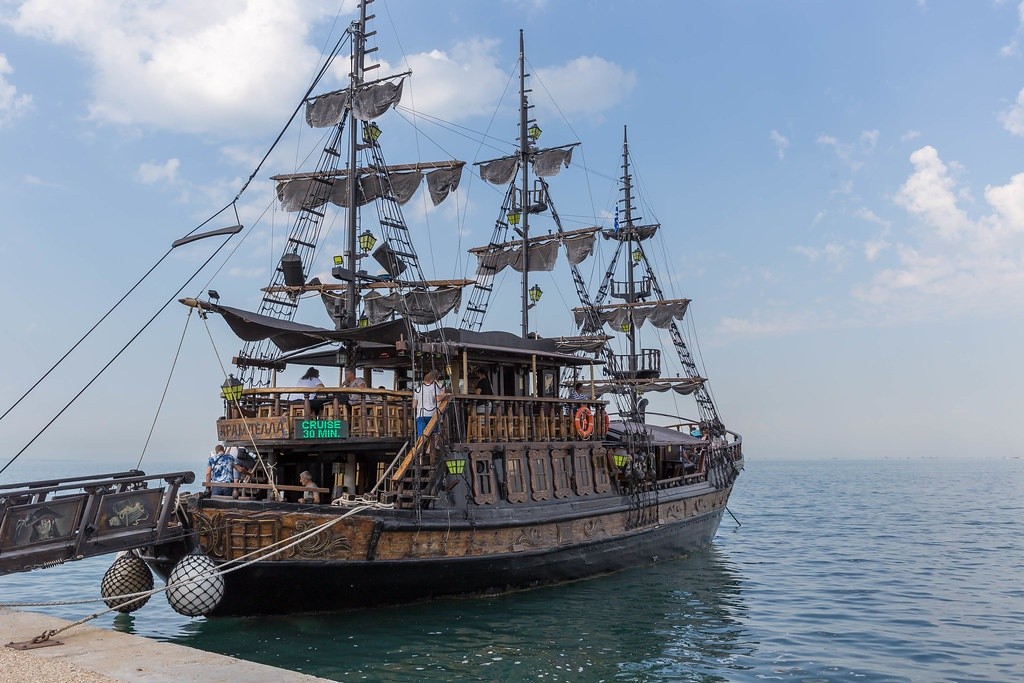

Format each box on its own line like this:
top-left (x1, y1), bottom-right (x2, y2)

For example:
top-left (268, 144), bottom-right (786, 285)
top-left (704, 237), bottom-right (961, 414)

top-left (575, 406), bottom-right (593, 437)
top-left (593, 411), bottom-right (610, 436)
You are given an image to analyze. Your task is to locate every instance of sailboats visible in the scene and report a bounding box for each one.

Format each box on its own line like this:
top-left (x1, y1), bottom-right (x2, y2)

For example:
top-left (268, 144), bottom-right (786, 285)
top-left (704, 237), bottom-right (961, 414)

top-left (123, 1), bottom-right (745, 615)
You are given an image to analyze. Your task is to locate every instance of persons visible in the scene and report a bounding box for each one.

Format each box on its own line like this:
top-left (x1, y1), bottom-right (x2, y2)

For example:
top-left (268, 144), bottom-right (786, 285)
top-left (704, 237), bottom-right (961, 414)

top-left (298, 471), bottom-right (320, 505)
top-left (205, 444), bottom-right (253, 495)
top-left (412, 369), bottom-right (452, 465)
top-left (288, 367), bottom-right (325, 415)
top-left (680, 427), bottom-right (723, 483)
top-left (333, 369), bottom-right (372, 415)
top-left (566, 383), bottom-right (591, 416)
top-left (475, 367), bottom-right (493, 415)
